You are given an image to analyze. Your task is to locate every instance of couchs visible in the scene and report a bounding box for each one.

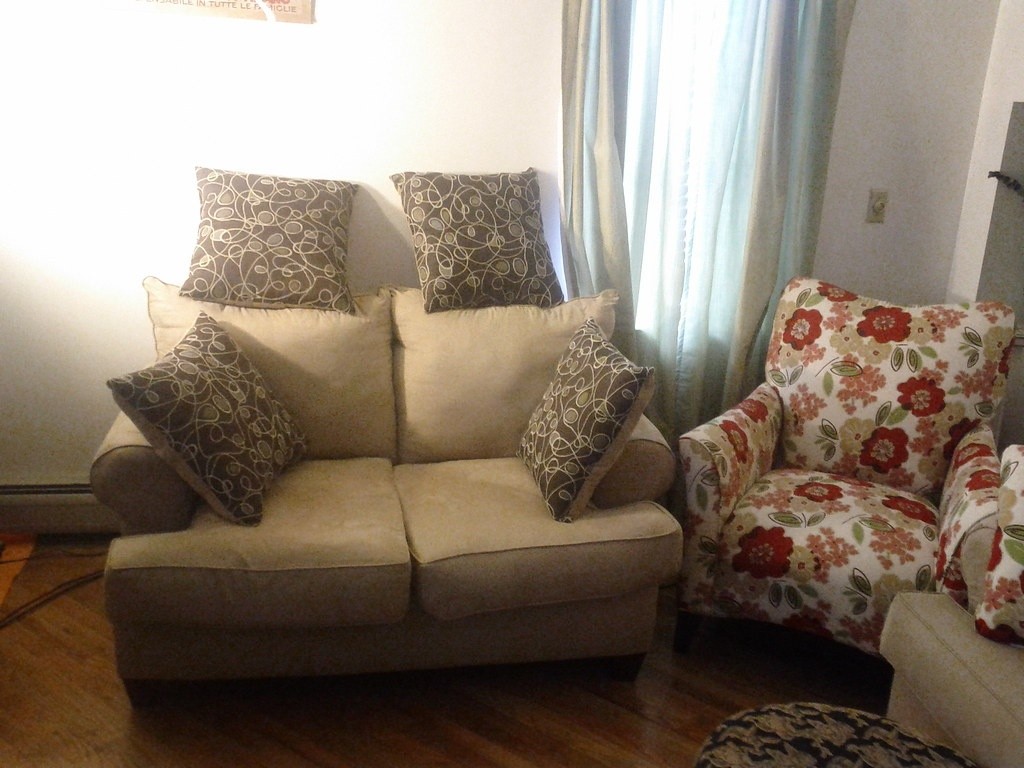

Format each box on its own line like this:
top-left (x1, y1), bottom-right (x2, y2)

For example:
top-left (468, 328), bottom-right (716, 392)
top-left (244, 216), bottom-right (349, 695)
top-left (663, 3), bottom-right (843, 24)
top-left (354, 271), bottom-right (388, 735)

top-left (87, 276), bottom-right (685, 713)
top-left (676, 275), bottom-right (1018, 681)
top-left (877, 592), bottom-right (1024, 768)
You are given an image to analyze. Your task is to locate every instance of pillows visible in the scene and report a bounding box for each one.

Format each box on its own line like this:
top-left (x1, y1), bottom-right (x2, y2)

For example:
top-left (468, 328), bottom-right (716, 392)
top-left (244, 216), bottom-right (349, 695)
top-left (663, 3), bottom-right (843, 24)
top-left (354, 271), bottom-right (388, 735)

top-left (106, 308), bottom-right (311, 528)
top-left (973, 443), bottom-right (1023, 647)
top-left (514, 316), bottom-right (657, 524)
top-left (177, 166), bottom-right (359, 318)
top-left (388, 166), bottom-right (565, 315)
